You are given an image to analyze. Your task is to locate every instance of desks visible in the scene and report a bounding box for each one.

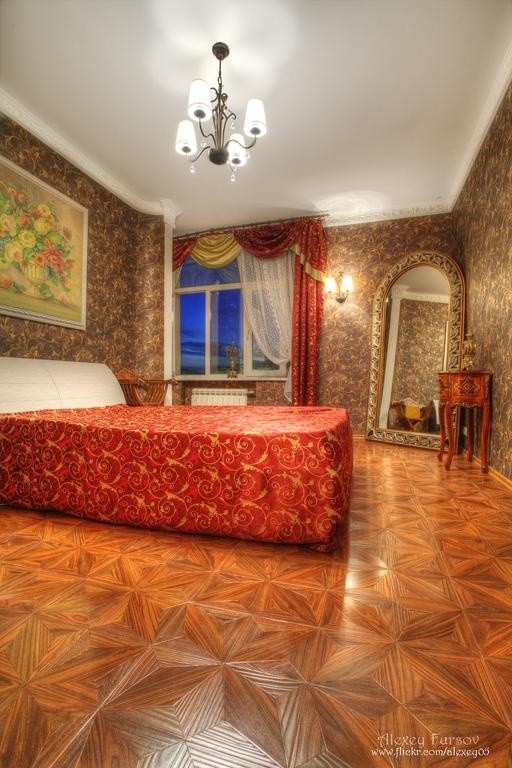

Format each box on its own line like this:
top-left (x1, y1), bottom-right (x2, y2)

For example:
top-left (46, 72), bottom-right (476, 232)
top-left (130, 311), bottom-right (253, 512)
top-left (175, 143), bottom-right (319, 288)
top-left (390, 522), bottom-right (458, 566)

top-left (437, 371), bottom-right (493, 474)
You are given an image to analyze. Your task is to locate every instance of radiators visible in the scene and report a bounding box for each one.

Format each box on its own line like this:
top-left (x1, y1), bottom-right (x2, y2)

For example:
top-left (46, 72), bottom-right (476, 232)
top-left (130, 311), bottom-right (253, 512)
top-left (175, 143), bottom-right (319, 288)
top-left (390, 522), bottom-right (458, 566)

top-left (191, 388), bottom-right (255, 406)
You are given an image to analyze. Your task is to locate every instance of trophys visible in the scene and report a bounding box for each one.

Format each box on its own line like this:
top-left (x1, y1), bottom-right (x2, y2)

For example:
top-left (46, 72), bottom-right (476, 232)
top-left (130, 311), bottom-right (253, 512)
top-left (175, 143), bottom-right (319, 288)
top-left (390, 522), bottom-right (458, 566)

top-left (225, 341), bottom-right (240, 378)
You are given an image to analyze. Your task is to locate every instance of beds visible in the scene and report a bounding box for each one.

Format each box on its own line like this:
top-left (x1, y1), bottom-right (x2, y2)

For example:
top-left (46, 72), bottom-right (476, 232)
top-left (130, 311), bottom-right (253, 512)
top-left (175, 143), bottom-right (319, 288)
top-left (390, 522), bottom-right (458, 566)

top-left (0, 357), bottom-right (353, 553)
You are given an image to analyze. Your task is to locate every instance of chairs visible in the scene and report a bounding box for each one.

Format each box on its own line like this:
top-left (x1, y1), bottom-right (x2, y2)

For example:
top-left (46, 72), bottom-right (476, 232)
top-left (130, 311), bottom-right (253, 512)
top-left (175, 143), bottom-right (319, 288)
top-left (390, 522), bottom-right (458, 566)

top-left (115, 367), bottom-right (177, 406)
top-left (390, 397), bottom-right (433, 432)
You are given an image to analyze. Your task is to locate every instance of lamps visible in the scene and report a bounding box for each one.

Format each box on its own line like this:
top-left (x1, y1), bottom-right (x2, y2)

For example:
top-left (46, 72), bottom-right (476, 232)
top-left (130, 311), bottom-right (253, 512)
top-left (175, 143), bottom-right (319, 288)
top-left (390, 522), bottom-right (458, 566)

top-left (323, 272), bottom-right (354, 302)
top-left (176, 42), bottom-right (269, 181)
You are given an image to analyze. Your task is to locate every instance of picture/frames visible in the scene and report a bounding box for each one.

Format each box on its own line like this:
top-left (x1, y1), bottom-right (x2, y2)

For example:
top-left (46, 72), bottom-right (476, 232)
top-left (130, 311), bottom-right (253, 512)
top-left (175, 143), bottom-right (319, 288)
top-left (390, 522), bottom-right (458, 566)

top-left (0, 155), bottom-right (89, 332)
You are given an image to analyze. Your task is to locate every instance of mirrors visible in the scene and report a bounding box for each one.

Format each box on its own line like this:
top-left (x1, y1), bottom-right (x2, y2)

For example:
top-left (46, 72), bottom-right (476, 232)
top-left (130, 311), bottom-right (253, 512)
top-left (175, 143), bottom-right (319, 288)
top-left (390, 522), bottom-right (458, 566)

top-left (365, 250), bottom-right (466, 455)
